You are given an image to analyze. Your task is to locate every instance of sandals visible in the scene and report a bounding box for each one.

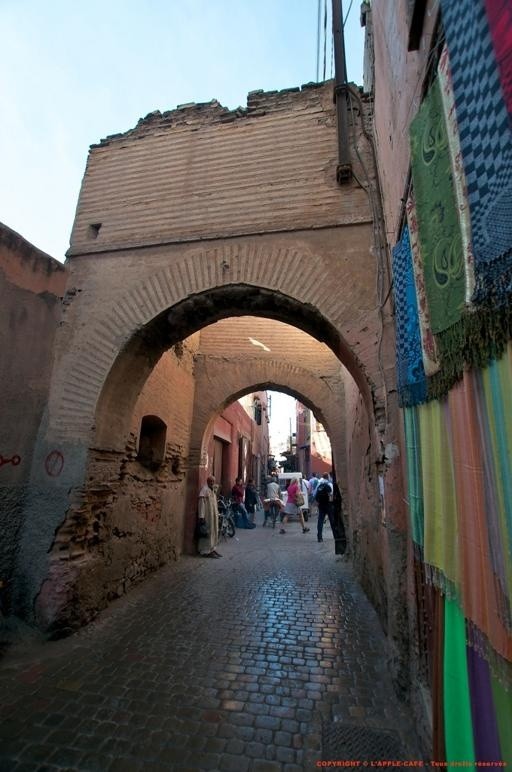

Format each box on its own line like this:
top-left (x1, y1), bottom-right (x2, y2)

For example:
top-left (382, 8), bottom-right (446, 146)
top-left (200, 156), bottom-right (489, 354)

top-left (302, 527), bottom-right (309, 533)
top-left (279, 528), bottom-right (286, 533)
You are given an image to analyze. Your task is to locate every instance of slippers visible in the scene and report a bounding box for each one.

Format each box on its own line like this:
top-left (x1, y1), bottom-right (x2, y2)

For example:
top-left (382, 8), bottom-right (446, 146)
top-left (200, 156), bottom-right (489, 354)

top-left (213, 551), bottom-right (221, 557)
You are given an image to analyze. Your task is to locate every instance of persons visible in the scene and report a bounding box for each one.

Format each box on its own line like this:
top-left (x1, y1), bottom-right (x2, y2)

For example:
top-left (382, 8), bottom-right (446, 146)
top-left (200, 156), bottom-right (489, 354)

top-left (198, 475), bottom-right (224, 558)
top-left (232, 471), bottom-right (333, 543)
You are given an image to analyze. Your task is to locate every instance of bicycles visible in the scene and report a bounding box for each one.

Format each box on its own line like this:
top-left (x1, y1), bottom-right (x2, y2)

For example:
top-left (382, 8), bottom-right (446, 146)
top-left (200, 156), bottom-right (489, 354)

top-left (216, 497), bottom-right (235, 536)
top-left (268, 502), bottom-right (278, 528)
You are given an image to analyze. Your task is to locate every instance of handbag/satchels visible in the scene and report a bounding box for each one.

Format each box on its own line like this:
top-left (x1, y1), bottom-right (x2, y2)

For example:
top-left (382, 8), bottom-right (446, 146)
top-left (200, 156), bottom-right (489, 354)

top-left (295, 494), bottom-right (304, 507)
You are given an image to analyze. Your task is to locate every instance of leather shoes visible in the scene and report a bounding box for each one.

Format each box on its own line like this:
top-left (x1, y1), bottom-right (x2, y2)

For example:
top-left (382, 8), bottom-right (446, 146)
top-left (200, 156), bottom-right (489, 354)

top-left (209, 552), bottom-right (219, 559)
top-left (318, 538), bottom-right (324, 542)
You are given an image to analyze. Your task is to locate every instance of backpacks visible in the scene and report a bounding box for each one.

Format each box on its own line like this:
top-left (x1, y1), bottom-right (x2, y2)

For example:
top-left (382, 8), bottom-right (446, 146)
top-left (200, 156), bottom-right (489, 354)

top-left (315, 482), bottom-right (331, 505)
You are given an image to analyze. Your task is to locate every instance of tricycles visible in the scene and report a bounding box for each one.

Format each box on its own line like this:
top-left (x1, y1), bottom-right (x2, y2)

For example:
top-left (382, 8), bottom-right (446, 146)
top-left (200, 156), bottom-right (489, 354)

top-left (278, 473), bottom-right (309, 522)
